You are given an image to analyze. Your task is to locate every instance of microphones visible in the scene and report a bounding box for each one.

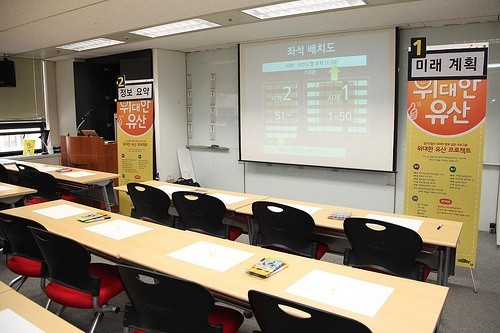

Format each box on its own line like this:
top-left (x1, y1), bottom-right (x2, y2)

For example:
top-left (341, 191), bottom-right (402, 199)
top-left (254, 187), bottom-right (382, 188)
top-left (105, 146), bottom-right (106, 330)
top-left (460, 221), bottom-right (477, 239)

top-left (81, 109), bottom-right (93, 119)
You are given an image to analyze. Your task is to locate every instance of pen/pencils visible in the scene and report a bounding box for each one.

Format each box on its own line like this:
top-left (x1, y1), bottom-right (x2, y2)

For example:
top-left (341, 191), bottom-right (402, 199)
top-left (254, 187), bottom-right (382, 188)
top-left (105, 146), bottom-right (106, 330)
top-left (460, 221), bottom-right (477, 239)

top-left (437, 222), bottom-right (446, 228)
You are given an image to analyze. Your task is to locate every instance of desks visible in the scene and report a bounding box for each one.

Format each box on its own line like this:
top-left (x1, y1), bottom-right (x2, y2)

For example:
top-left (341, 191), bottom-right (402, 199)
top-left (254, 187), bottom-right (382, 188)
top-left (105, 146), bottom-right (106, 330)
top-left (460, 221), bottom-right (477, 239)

top-left (0, 161), bottom-right (463, 333)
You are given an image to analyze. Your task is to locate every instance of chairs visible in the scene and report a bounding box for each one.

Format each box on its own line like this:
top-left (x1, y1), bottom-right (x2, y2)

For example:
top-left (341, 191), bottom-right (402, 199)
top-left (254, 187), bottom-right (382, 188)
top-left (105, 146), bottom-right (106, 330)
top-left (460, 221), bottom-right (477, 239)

top-left (0, 162), bottom-right (430, 333)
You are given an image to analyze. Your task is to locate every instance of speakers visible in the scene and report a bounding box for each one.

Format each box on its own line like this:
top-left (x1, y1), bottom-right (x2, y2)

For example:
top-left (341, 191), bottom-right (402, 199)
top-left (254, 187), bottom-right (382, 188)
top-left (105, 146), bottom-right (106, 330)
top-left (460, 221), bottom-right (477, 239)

top-left (0, 59), bottom-right (16, 87)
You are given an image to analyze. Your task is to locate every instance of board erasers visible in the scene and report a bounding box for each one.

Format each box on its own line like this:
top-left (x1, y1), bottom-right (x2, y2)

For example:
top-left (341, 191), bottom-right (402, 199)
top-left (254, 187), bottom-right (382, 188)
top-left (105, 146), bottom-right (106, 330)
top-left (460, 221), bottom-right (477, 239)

top-left (211, 145), bottom-right (220, 147)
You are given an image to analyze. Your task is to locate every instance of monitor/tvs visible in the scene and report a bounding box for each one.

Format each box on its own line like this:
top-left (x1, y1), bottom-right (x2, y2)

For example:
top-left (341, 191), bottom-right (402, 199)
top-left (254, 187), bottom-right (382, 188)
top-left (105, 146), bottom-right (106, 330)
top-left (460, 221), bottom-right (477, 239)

top-left (82, 130), bottom-right (98, 136)
